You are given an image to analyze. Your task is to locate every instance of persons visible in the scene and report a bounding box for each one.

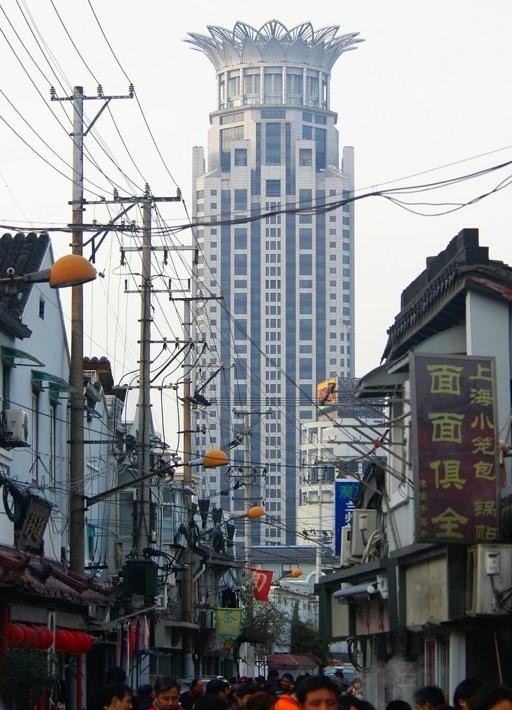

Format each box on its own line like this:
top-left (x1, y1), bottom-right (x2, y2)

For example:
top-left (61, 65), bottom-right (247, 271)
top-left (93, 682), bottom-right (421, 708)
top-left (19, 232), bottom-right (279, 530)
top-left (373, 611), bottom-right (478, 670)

top-left (47, 667), bottom-right (512, 710)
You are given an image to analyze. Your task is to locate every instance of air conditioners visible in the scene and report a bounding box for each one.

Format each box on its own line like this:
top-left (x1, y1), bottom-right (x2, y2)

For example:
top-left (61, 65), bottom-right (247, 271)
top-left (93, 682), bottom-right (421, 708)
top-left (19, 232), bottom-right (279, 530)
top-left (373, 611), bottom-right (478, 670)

top-left (338, 508), bottom-right (379, 569)
top-left (463, 542), bottom-right (511, 620)
top-left (6, 408), bottom-right (30, 443)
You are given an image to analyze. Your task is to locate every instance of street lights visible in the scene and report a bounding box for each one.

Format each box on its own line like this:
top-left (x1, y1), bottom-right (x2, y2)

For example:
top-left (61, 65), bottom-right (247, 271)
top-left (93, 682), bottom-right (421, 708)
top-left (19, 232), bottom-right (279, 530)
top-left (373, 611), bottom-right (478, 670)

top-left (0, 254), bottom-right (97, 289)
